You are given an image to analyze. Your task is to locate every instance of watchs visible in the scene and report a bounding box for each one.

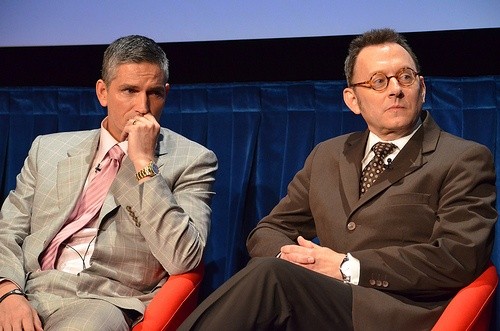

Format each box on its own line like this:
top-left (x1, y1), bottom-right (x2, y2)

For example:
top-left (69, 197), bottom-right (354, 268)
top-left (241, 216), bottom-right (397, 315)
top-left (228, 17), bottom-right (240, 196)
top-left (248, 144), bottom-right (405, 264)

top-left (135, 160), bottom-right (159, 182)
top-left (338, 254), bottom-right (350, 283)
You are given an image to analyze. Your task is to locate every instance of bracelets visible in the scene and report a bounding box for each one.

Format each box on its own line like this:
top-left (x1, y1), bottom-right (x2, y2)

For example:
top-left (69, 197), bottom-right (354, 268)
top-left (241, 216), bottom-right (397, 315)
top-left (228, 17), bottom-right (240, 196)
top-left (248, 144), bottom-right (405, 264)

top-left (0, 289), bottom-right (27, 303)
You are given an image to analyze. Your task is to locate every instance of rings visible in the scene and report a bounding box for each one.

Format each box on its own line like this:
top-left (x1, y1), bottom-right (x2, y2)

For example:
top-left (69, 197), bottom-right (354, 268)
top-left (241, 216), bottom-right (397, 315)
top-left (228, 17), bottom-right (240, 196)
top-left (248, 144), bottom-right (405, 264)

top-left (133, 120), bottom-right (138, 125)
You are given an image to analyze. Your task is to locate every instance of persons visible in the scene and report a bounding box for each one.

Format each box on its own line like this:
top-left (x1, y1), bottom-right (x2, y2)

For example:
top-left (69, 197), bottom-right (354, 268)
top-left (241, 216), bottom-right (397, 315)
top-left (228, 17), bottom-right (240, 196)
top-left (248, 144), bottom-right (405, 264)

top-left (176, 27), bottom-right (500, 331)
top-left (0, 35), bottom-right (219, 331)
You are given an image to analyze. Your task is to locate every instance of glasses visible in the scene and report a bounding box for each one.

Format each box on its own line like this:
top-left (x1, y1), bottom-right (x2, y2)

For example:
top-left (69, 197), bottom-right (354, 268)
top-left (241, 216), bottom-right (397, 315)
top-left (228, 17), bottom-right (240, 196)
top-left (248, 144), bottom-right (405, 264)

top-left (349, 68), bottom-right (420, 91)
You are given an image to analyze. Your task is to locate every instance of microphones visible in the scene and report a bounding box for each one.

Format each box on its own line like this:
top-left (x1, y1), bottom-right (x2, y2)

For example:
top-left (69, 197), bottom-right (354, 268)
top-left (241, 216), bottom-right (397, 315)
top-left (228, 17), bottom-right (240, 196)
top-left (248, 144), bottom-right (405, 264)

top-left (95, 163), bottom-right (101, 172)
top-left (383, 158), bottom-right (393, 170)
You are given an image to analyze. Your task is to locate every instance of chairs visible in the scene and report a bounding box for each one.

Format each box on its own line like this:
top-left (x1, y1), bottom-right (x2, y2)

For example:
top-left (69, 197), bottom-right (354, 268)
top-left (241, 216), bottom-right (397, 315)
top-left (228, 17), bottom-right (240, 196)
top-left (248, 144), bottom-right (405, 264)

top-left (431, 261), bottom-right (500, 331)
top-left (130, 257), bottom-right (207, 331)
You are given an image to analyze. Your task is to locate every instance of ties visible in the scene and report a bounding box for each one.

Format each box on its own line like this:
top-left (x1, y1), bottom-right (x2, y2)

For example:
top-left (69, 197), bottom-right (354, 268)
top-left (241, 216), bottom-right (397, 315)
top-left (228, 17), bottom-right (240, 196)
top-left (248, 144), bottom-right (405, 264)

top-left (357, 140), bottom-right (399, 196)
top-left (38, 145), bottom-right (125, 271)
